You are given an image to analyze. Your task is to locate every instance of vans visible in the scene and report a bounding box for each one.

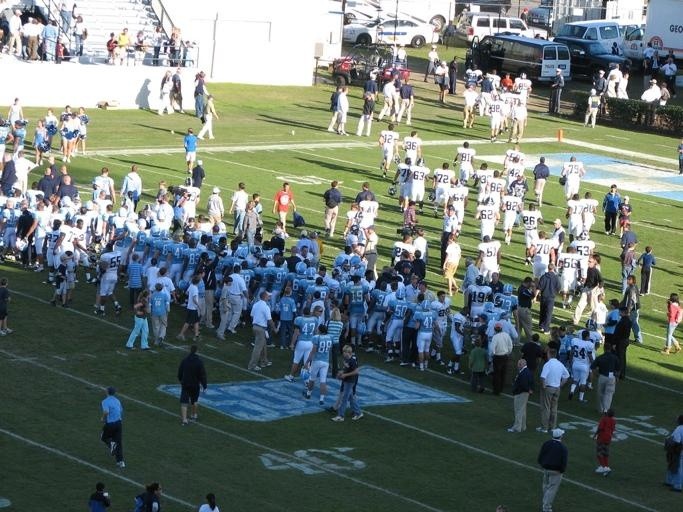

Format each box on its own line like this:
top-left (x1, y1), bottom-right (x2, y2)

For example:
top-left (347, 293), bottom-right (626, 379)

top-left (455, 0), bottom-right (682, 83)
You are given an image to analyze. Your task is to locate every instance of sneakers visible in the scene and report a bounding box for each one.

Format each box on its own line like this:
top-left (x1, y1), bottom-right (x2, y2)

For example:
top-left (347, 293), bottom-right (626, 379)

top-left (115, 305), bottom-right (122, 315)
top-left (323, 405), bottom-right (364, 422)
top-left (260, 361), bottom-right (273, 367)
top-left (49, 295), bottom-right (72, 308)
top-left (569, 379), bottom-right (594, 405)
top-left (121, 317), bottom-right (289, 351)
top-left (0, 329), bottom-right (15, 336)
top-left (284, 374), bottom-right (296, 383)
top-left (181, 414), bottom-right (198, 425)
top-left (350, 344), bottom-right (461, 375)
top-left (302, 390), bottom-right (311, 399)
top-left (1, 251), bottom-right (43, 273)
top-left (21, 252), bottom-right (22, 255)
top-left (93, 310), bottom-right (106, 316)
top-left (319, 401), bottom-right (324, 405)
top-left (595, 466), bottom-right (611, 474)
top-left (248, 366), bottom-right (262, 371)
top-left (115, 461), bottom-right (125, 468)
top-left (110, 441), bottom-right (117, 455)
top-left (83, 272), bottom-right (101, 283)
top-left (535, 426), bottom-right (556, 433)
top-left (506, 425), bottom-right (527, 432)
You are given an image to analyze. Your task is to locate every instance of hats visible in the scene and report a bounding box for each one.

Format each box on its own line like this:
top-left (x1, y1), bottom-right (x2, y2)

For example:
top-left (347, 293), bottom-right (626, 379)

top-left (552, 429), bottom-right (565, 437)
top-left (212, 187), bottom-right (221, 193)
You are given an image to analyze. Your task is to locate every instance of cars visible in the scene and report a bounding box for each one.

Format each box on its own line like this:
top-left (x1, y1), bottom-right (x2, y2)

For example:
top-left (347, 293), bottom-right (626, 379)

top-left (341, 0), bottom-right (436, 51)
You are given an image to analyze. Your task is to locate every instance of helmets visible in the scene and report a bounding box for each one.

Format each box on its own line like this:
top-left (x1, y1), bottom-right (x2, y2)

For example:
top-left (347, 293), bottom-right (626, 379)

top-left (421, 300), bottom-right (430, 312)
top-left (296, 262), bottom-right (307, 275)
top-left (235, 247), bottom-right (249, 259)
top-left (585, 319), bottom-right (597, 330)
top-left (305, 267), bottom-right (317, 278)
top-left (483, 302), bottom-right (494, 313)
top-left (503, 284), bottom-right (512, 295)
top-left (395, 288), bottom-right (405, 299)
top-left (334, 255), bottom-right (365, 277)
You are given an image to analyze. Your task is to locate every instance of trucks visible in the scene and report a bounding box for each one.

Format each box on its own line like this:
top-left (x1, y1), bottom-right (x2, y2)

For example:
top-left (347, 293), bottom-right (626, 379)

top-left (377, 0), bottom-right (455, 33)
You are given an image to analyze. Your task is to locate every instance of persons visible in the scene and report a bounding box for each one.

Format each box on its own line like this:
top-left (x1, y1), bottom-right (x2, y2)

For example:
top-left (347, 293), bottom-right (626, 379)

top-left (538, 428), bottom-right (568, 512)
top-left (134, 482), bottom-right (161, 512)
top-left (592, 408), bottom-right (616, 477)
top-left (664, 414), bottom-right (683, 492)
top-left (496, 505), bottom-right (509, 512)
top-left (99, 387), bottom-right (126, 468)
top-left (88, 482), bottom-right (112, 512)
top-left (199, 493), bottom-right (219, 512)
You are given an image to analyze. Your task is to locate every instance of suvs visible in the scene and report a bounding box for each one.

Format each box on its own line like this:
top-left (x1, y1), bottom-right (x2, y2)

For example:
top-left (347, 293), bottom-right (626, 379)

top-left (328, 41), bottom-right (411, 91)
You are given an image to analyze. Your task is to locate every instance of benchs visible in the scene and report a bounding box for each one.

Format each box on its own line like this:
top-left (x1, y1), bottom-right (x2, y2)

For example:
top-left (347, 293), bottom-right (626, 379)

top-left (0, 0), bottom-right (179, 66)
